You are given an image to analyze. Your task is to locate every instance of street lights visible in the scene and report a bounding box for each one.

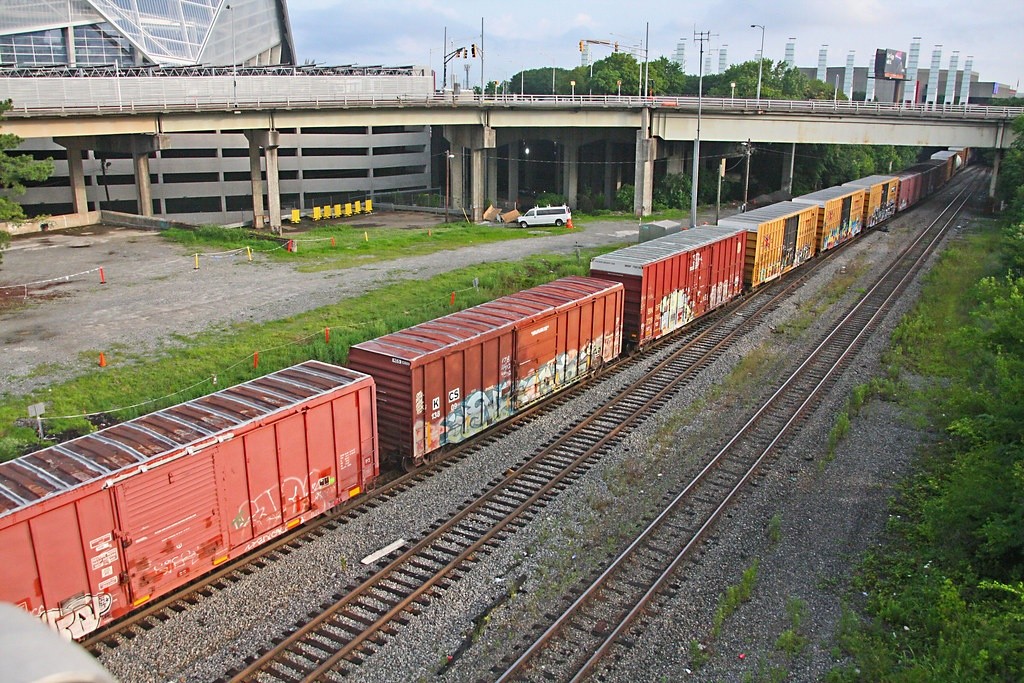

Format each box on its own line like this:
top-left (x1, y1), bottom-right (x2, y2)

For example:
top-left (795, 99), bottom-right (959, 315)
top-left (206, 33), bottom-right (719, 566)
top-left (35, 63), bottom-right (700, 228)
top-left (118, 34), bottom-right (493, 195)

top-left (101, 162), bottom-right (113, 211)
top-left (539, 52), bottom-right (555, 95)
top-left (740, 142), bottom-right (751, 213)
top-left (610, 32), bottom-right (643, 100)
top-left (226, 6), bottom-right (236, 108)
top-left (509, 61), bottom-right (524, 99)
top-left (752, 24), bottom-right (766, 103)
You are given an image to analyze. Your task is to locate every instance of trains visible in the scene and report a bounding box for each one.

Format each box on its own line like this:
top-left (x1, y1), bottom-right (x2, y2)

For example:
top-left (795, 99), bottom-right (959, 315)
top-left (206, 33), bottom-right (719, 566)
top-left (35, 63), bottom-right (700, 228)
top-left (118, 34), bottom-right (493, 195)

top-left (1, 138), bottom-right (976, 643)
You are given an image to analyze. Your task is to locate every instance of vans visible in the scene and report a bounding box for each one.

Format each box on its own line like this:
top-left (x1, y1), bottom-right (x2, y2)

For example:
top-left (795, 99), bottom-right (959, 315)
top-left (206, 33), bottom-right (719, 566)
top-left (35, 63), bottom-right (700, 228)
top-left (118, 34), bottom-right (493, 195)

top-left (518, 205), bottom-right (572, 228)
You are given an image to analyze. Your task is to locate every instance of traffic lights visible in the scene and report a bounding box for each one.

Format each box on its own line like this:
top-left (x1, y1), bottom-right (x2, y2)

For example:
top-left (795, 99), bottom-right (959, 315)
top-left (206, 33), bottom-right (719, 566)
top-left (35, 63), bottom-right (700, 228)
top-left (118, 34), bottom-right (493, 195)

top-left (463, 49), bottom-right (468, 59)
top-left (471, 44), bottom-right (477, 57)
top-left (614, 42), bottom-right (618, 52)
top-left (579, 41), bottom-right (583, 52)
top-left (456, 52), bottom-right (461, 57)
